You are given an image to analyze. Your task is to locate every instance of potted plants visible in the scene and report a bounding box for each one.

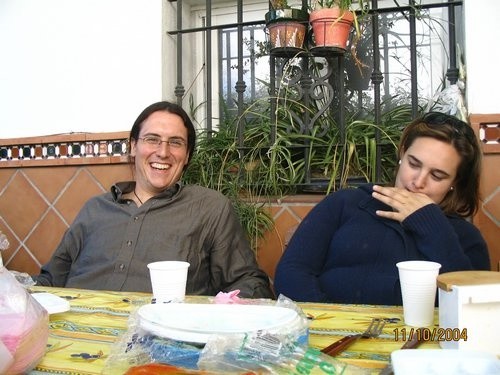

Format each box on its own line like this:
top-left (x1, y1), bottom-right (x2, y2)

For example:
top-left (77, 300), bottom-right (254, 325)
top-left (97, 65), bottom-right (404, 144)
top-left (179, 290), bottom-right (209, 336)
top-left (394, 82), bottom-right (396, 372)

top-left (180, 0), bottom-right (454, 261)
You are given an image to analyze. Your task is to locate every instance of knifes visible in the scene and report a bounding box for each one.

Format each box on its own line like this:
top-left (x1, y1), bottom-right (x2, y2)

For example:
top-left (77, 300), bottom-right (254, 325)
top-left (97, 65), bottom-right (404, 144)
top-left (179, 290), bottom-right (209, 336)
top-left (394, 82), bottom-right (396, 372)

top-left (380, 327), bottom-right (429, 375)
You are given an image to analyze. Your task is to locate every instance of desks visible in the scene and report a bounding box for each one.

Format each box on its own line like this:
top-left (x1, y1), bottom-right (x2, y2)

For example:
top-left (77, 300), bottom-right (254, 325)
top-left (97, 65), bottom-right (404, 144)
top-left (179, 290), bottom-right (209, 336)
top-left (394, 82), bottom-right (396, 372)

top-left (29, 285), bottom-right (441, 375)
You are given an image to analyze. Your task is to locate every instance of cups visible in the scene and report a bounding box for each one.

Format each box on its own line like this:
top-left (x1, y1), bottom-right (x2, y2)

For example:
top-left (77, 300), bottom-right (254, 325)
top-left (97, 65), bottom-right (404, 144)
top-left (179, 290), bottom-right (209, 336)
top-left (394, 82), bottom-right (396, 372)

top-left (396, 261), bottom-right (442, 327)
top-left (148, 260), bottom-right (190, 303)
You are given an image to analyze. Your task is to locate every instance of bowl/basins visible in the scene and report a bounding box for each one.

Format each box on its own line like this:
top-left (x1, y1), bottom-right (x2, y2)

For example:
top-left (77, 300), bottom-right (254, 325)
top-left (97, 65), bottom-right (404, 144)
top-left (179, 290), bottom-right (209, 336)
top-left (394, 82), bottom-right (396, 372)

top-left (391, 349), bottom-right (500, 375)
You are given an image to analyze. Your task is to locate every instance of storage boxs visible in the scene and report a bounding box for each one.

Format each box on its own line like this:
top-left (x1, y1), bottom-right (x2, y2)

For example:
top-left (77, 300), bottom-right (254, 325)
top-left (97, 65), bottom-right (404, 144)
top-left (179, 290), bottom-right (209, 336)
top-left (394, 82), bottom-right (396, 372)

top-left (436, 272), bottom-right (500, 351)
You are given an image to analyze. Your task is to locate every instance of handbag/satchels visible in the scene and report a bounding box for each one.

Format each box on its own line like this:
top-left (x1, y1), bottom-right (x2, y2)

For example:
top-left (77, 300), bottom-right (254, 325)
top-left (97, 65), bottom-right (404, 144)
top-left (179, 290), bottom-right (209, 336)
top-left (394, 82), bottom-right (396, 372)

top-left (0, 258), bottom-right (49, 375)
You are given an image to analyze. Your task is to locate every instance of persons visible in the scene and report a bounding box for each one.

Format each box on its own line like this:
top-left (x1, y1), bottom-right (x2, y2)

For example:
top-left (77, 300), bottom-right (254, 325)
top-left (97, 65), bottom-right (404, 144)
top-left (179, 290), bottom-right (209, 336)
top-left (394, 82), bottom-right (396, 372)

top-left (30, 102), bottom-right (274, 302)
top-left (271, 112), bottom-right (490, 307)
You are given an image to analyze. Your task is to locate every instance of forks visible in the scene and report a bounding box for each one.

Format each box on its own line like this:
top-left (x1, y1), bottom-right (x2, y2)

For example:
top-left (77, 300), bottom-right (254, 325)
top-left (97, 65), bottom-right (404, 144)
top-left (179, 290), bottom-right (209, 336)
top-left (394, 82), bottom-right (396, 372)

top-left (321, 318), bottom-right (386, 355)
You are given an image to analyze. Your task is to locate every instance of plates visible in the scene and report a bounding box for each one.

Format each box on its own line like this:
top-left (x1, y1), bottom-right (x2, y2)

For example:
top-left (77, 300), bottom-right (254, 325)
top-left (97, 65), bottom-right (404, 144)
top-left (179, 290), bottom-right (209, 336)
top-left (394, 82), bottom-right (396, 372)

top-left (137, 303), bottom-right (300, 345)
top-left (31, 293), bottom-right (70, 314)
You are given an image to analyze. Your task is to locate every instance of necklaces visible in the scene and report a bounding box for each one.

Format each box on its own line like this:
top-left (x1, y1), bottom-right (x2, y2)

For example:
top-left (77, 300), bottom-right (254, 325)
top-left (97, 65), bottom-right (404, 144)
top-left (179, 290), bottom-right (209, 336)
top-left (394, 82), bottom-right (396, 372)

top-left (133, 188), bottom-right (143, 205)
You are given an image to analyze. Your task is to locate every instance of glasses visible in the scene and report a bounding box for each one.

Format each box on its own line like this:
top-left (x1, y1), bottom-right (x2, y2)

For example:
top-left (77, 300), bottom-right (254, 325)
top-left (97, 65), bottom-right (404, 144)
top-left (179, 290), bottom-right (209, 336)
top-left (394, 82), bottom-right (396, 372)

top-left (421, 112), bottom-right (475, 145)
top-left (136, 135), bottom-right (186, 148)
